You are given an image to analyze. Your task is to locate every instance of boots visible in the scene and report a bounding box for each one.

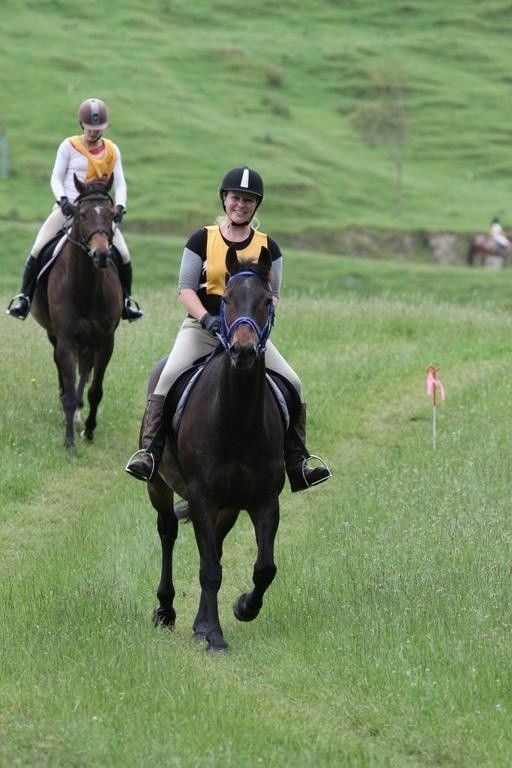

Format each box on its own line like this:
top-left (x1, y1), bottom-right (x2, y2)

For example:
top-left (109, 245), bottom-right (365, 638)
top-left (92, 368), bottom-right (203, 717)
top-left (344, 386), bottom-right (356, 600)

top-left (11, 255), bottom-right (39, 317)
top-left (128, 393), bottom-right (165, 480)
top-left (121, 262), bottom-right (142, 318)
top-left (285, 401), bottom-right (329, 492)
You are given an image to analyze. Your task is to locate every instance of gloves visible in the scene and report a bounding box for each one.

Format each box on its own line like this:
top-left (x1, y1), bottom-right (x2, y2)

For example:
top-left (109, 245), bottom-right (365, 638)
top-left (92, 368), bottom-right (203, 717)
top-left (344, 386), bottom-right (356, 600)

top-left (200, 313), bottom-right (220, 336)
top-left (57, 196), bottom-right (73, 215)
top-left (114, 205), bottom-right (124, 222)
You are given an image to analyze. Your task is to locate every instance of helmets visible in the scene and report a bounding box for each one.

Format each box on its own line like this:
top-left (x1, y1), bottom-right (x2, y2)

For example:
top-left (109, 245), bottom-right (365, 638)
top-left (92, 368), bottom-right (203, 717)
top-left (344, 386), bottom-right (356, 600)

top-left (221, 168), bottom-right (263, 198)
top-left (79, 99), bottom-right (109, 130)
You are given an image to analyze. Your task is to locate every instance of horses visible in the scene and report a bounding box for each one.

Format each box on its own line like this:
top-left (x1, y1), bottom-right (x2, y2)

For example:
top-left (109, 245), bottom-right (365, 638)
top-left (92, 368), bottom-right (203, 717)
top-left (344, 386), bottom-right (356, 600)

top-left (27, 171), bottom-right (126, 467)
top-left (135, 245), bottom-right (295, 655)
top-left (467, 234), bottom-right (512, 268)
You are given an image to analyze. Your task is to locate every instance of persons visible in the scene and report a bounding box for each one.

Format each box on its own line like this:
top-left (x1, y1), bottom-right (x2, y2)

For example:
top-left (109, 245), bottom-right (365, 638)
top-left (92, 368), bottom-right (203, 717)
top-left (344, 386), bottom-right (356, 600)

top-left (4, 98), bottom-right (148, 320)
top-left (489, 216), bottom-right (510, 257)
top-left (124, 166), bottom-right (331, 493)
